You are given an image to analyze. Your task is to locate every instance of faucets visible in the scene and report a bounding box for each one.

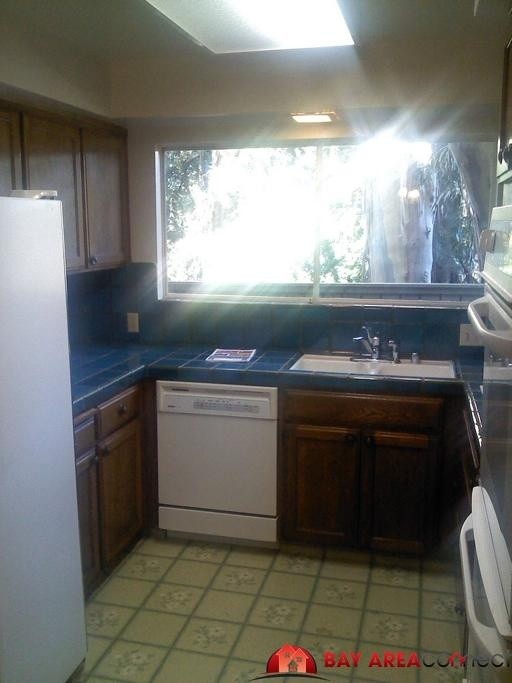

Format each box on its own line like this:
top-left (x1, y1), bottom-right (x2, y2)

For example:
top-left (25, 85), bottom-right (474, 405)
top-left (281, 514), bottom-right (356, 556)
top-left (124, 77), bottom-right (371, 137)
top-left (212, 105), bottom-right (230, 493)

top-left (353, 327), bottom-right (380, 359)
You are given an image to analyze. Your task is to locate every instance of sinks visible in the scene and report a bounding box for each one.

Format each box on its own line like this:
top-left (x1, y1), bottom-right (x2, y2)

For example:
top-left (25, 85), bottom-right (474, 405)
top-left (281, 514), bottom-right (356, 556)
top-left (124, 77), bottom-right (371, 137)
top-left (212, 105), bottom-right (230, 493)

top-left (289, 354), bottom-right (455, 379)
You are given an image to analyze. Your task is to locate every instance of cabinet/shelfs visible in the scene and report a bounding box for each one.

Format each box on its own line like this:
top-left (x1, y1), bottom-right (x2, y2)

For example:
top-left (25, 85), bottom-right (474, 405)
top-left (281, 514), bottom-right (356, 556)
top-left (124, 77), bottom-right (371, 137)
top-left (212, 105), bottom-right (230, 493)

top-left (0, 98), bottom-right (22, 194)
top-left (73, 379), bottom-right (146, 600)
top-left (12, 111), bottom-right (131, 282)
top-left (282, 388), bottom-right (447, 560)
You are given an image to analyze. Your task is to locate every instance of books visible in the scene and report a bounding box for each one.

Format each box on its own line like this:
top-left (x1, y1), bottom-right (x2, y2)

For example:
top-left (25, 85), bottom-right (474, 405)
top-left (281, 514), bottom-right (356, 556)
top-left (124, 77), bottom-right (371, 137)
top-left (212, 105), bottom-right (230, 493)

top-left (205, 346), bottom-right (258, 364)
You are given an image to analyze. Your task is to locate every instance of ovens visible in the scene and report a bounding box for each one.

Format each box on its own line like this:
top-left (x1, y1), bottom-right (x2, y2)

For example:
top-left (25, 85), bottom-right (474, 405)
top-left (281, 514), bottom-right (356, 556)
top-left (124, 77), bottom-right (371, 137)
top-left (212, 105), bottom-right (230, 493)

top-left (153, 378), bottom-right (280, 551)
top-left (452, 30), bottom-right (510, 661)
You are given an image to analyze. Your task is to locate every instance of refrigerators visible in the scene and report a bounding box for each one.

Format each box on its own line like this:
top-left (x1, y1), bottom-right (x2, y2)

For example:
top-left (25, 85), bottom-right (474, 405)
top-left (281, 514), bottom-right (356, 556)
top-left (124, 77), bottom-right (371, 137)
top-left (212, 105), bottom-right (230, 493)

top-left (0, 184), bottom-right (90, 681)
